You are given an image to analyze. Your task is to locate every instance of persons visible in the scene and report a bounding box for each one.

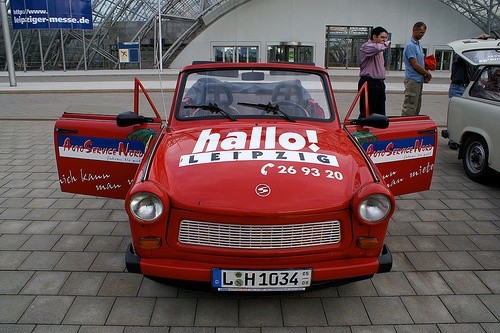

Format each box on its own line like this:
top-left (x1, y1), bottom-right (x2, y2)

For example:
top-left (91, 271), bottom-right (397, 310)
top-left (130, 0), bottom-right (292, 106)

top-left (448, 34), bottom-right (490, 150)
top-left (402, 22), bottom-right (432, 116)
top-left (358, 27), bottom-right (391, 120)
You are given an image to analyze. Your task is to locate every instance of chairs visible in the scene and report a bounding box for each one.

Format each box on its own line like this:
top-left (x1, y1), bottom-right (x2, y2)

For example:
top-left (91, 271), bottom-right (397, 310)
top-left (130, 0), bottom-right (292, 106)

top-left (264, 82), bottom-right (314, 117)
top-left (480, 70), bottom-right (500, 101)
top-left (190, 81), bottom-right (234, 115)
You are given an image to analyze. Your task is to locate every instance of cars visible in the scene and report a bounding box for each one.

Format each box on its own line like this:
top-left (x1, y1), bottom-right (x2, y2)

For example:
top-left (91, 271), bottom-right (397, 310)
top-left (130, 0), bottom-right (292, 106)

top-left (53, 60), bottom-right (439, 299)
top-left (441, 37), bottom-right (500, 182)
